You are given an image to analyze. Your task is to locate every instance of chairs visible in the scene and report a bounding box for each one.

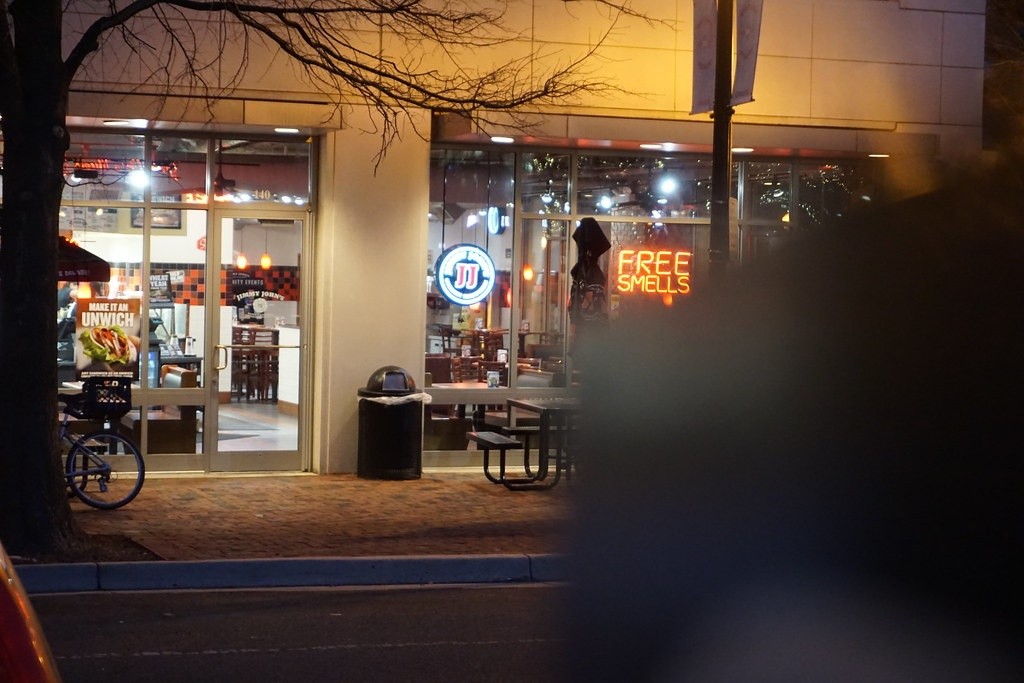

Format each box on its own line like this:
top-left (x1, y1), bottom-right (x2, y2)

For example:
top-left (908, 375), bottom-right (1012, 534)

top-left (441, 323), bottom-right (564, 386)
top-left (232, 325), bottom-right (279, 402)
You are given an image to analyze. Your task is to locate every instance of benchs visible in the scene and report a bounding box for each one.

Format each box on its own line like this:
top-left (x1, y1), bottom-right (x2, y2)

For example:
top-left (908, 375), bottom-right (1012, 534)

top-left (120, 362), bottom-right (196, 453)
top-left (466, 425), bottom-right (570, 481)
top-left (58, 411), bottom-right (108, 455)
top-left (484, 366), bottom-right (565, 449)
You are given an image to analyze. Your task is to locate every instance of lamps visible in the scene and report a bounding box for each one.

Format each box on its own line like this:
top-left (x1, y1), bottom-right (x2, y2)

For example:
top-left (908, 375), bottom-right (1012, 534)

top-left (237, 220), bottom-right (247, 270)
top-left (261, 224), bottom-right (271, 269)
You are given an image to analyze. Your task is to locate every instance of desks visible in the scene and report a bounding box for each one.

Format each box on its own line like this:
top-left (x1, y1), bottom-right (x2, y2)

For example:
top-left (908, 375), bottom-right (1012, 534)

top-left (461, 329), bottom-right (510, 335)
top-left (506, 395), bottom-right (578, 484)
top-left (504, 362), bottom-right (531, 368)
top-left (518, 330), bottom-right (546, 357)
top-left (62, 380), bottom-right (140, 390)
top-left (431, 382), bottom-right (509, 450)
top-left (453, 355), bottom-right (482, 363)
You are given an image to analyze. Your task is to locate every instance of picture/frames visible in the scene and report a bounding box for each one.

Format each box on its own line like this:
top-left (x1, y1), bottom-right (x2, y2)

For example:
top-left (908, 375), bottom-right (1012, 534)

top-left (130, 194), bottom-right (181, 229)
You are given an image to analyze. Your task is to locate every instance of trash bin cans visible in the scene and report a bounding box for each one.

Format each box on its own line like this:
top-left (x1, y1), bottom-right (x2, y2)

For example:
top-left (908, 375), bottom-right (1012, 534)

top-left (355, 366), bottom-right (427, 483)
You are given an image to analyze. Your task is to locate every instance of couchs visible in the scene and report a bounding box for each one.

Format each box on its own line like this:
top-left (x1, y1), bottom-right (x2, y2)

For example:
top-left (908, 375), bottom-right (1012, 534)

top-left (425, 352), bottom-right (456, 416)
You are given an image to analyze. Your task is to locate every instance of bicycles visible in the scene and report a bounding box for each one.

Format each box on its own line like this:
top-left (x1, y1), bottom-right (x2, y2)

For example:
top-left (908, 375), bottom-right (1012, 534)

top-left (58, 393), bottom-right (145, 510)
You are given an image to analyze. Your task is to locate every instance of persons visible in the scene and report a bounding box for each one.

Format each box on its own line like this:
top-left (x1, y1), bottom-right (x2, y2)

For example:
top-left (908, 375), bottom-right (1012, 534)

top-left (58, 282), bottom-right (79, 311)
